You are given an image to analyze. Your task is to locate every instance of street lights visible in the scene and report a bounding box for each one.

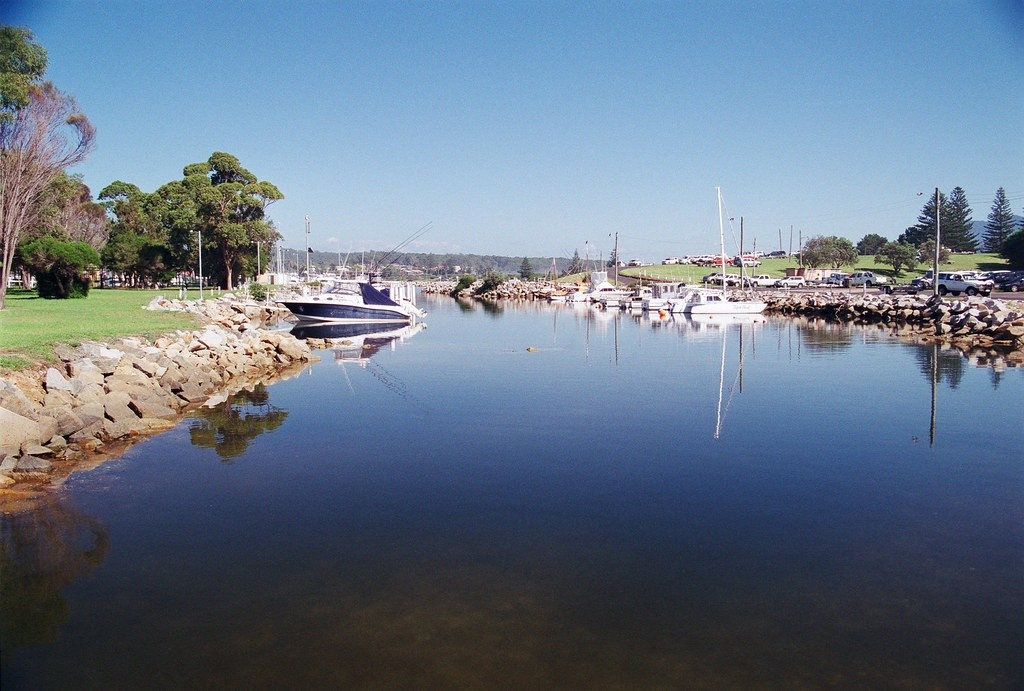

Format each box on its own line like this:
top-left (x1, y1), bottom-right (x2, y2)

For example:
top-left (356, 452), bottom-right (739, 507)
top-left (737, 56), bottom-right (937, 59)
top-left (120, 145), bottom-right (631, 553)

top-left (251, 240), bottom-right (260, 282)
top-left (190, 230), bottom-right (203, 301)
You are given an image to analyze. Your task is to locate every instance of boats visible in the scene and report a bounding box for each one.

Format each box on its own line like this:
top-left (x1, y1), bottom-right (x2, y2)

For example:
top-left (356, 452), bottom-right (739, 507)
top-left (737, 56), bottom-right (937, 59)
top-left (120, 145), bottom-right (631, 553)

top-left (268, 220), bottom-right (432, 323)
top-left (288, 319), bottom-right (427, 367)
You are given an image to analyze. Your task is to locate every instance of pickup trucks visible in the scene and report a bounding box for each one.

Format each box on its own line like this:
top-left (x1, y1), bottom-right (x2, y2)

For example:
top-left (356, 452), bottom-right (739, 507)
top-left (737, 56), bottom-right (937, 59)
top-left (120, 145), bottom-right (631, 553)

top-left (748, 275), bottom-right (781, 288)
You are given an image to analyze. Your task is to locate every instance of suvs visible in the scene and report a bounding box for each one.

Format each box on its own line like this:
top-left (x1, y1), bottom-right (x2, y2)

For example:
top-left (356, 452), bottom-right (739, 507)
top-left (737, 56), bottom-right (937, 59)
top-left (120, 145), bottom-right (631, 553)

top-left (739, 251), bottom-right (758, 259)
top-left (937, 272), bottom-right (994, 297)
top-left (692, 255), bottom-right (715, 262)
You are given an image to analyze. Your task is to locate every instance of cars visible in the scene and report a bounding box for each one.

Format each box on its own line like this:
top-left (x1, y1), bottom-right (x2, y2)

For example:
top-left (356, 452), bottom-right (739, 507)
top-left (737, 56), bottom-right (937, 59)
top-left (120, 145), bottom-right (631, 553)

top-left (737, 258), bottom-right (763, 268)
top-left (998, 275), bottom-right (1024, 292)
top-left (978, 271), bottom-right (1024, 285)
top-left (789, 249), bottom-right (801, 256)
top-left (849, 272), bottom-right (888, 288)
top-left (728, 276), bottom-right (750, 287)
top-left (628, 259), bottom-right (641, 267)
top-left (678, 255), bottom-right (693, 265)
top-left (703, 272), bottom-right (718, 285)
top-left (716, 274), bottom-right (738, 286)
top-left (662, 257), bottom-right (679, 265)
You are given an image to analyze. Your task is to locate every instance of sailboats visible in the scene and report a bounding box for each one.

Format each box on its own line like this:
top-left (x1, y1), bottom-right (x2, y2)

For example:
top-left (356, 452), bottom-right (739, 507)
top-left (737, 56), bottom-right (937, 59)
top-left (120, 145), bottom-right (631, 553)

top-left (532, 184), bottom-right (769, 315)
top-left (687, 315), bottom-right (767, 439)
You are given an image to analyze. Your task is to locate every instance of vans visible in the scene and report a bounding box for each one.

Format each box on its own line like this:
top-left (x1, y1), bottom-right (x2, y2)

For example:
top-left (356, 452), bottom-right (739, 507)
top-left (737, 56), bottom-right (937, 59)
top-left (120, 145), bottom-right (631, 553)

top-left (829, 273), bottom-right (850, 284)
top-left (773, 276), bottom-right (806, 288)
top-left (768, 251), bottom-right (787, 258)
